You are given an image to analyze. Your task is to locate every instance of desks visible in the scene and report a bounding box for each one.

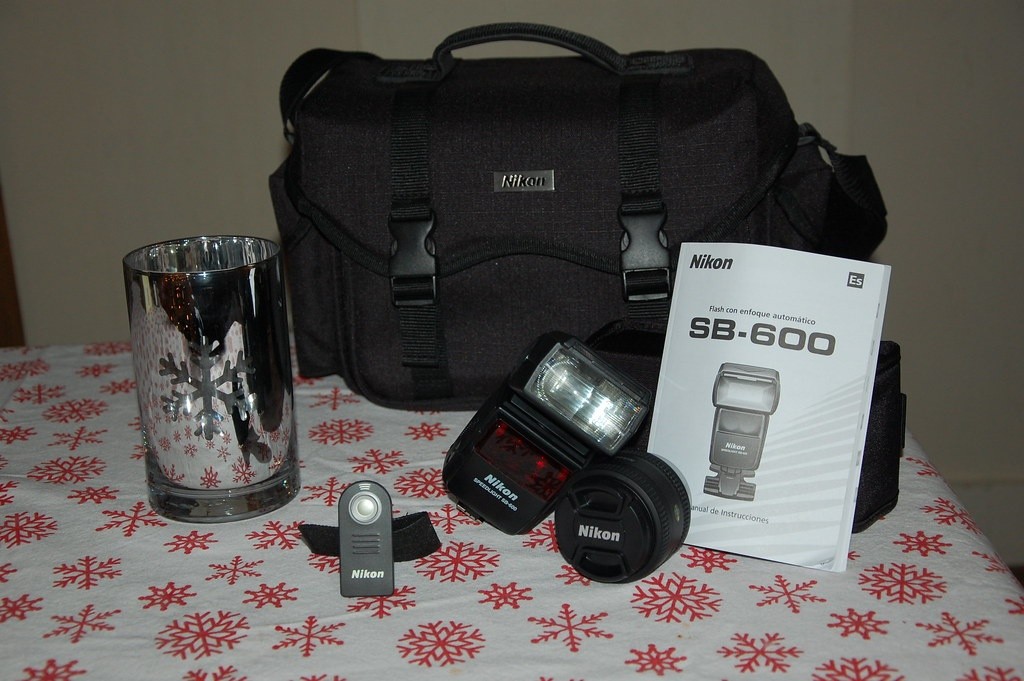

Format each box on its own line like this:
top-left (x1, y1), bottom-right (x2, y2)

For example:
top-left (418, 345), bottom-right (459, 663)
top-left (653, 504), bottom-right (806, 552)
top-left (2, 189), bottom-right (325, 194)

top-left (0, 342), bottom-right (1024, 681)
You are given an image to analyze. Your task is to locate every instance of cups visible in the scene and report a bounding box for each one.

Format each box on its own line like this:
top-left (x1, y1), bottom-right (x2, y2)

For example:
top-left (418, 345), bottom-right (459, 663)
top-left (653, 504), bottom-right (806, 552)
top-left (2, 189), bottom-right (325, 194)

top-left (122, 235), bottom-right (301, 525)
